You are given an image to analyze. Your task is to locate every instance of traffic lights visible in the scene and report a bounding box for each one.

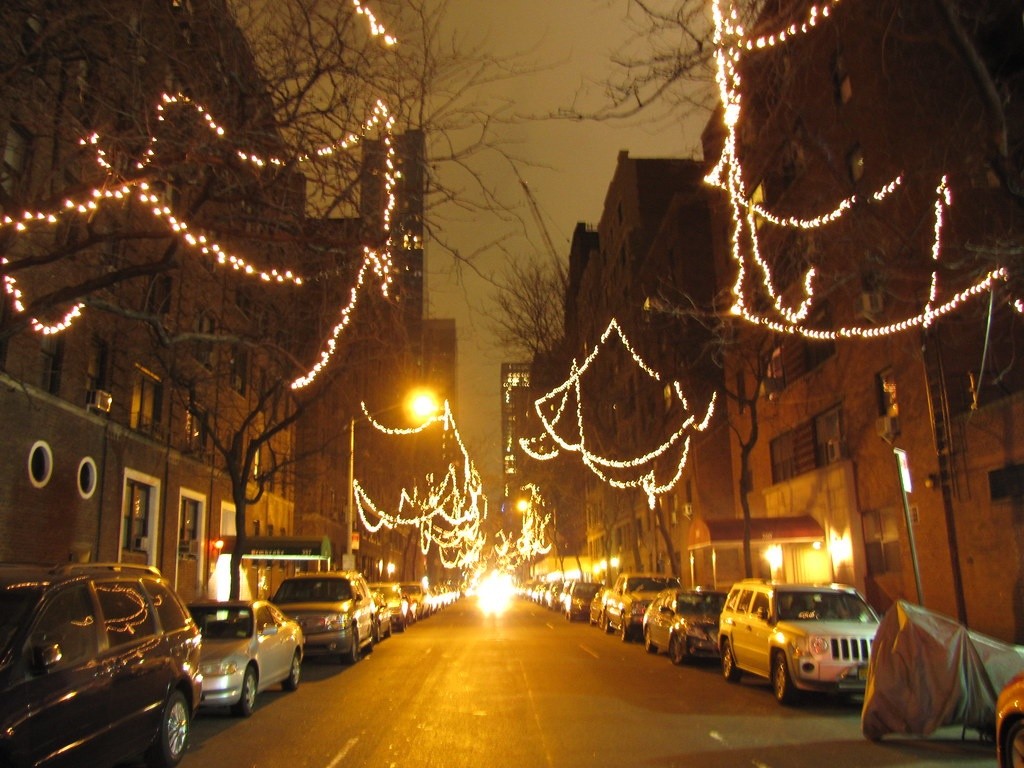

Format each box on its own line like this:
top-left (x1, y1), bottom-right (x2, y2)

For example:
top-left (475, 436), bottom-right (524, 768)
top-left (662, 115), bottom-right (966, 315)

top-left (873, 365), bottom-right (898, 423)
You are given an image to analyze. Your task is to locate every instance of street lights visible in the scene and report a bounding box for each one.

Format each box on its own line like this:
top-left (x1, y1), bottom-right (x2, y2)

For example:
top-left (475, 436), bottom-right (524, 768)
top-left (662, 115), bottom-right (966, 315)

top-left (345, 392), bottom-right (434, 555)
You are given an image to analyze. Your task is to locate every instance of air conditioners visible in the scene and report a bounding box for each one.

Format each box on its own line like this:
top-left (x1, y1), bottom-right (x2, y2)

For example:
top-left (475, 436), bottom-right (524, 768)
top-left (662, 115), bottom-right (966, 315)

top-left (682, 503), bottom-right (693, 515)
top-left (86, 389), bottom-right (113, 412)
top-left (162, 313), bottom-right (174, 333)
top-left (825, 439), bottom-right (842, 462)
top-left (758, 377), bottom-right (782, 397)
top-left (878, 415), bottom-right (899, 438)
top-left (852, 290), bottom-right (885, 320)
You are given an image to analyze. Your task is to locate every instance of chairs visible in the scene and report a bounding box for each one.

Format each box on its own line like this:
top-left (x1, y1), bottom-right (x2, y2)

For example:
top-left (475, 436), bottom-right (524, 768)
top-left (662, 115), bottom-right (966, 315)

top-left (813, 601), bottom-right (830, 615)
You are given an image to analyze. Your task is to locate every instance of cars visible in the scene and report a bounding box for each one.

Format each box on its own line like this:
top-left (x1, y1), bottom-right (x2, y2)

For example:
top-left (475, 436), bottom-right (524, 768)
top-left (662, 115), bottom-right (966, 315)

top-left (368, 581), bottom-right (409, 633)
top-left (183, 600), bottom-right (304, 719)
top-left (642, 587), bottom-right (729, 666)
top-left (402, 579), bottom-right (475, 623)
top-left (514, 577), bottom-right (609, 630)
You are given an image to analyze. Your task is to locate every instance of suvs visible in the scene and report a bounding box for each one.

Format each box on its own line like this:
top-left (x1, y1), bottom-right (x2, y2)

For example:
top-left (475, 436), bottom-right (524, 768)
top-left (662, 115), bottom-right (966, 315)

top-left (0, 563), bottom-right (204, 768)
top-left (716, 577), bottom-right (882, 706)
top-left (606, 571), bottom-right (684, 642)
top-left (266, 568), bottom-right (379, 666)
